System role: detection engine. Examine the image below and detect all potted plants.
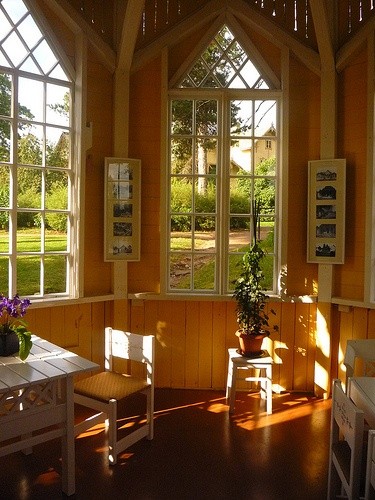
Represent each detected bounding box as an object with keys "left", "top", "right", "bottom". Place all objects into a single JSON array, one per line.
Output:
[{"left": 229, "top": 242, "right": 279, "bottom": 359}]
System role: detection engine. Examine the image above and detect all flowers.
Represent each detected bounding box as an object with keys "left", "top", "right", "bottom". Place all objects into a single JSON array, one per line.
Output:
[{"left": 0, "top": 292, "right": 33, "bottom": 361}]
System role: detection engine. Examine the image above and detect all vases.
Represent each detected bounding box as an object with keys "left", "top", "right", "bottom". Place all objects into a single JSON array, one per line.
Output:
[{"left": 0, "top": 332, "right": 19, "bottom": 357}]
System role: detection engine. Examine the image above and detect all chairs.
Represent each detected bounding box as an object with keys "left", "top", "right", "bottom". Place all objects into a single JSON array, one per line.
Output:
[
  {"left": 327, "top": 378, "right": 375, "bottom": 500},
  {"left": 344, "top": 339, "right": 375, "bottom": 441},
  {"left": 364, "top": 429, "right": 375, "bottom": 500},
  {"left": 73, "top": 327, "right": 155, "bottom": 466}
]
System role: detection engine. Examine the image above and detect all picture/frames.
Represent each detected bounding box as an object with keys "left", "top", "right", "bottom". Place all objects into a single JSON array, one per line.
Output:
[
  {"left": 104, "top": 156, "right": 141, "bottom": 262},
  {"left": 306, "top": 159, "right": 345, "bottom": 264}
]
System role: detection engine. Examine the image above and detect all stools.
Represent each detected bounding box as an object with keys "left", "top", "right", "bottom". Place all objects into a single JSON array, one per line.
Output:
[{"left": 226, "top": 348, "right": 273, "bottom": 416}]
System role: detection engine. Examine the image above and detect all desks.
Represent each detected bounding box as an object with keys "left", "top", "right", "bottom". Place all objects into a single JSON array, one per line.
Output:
[
  {"left": 0, "top": 334, "right": 100, "bottom": 496},
  {"left": 341, "top": 376, "right": 375, "bottom": 496}
]
